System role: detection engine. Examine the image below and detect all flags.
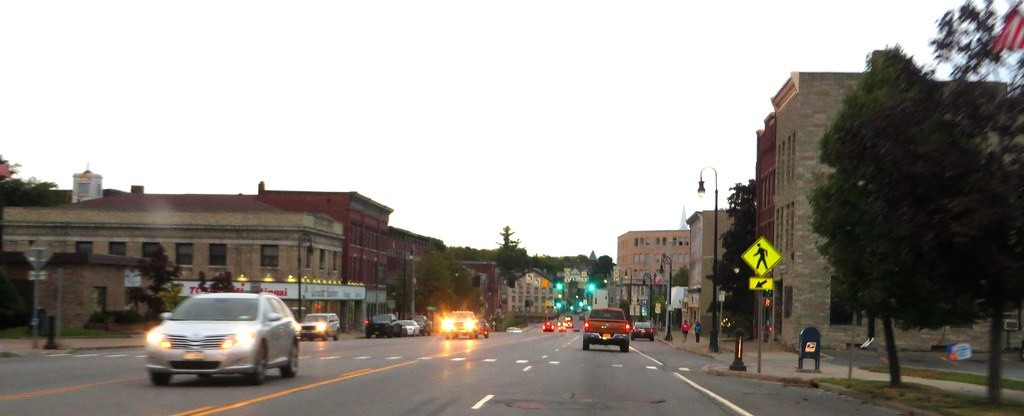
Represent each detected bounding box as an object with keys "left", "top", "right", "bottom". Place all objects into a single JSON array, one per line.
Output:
[{"left": 987, "top": 10, "right": 1024, "bottom": 53}]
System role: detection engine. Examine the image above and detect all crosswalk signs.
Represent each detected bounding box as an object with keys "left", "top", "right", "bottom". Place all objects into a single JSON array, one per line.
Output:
[{"left": 742, "top": 235, "right": 782, "bottom": 278}]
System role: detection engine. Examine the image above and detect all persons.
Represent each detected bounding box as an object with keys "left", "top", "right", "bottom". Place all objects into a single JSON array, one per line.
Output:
[
  {"left": 694, "top": 321, "right": 702, "bottom": 343},
  {"left": 680, "top": 320, "right": 690, "bottom": 344}
]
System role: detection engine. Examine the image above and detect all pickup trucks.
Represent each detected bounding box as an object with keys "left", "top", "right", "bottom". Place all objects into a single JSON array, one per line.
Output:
[{"left": 581, "top": 307, "right": 630, "bottom": 352}]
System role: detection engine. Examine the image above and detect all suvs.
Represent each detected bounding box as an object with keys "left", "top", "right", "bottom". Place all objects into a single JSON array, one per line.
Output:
[{"left": 299, "top": 312, "right": 340, "bottom": 341}]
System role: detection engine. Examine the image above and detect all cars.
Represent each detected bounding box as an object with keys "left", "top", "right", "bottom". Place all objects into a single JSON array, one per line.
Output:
[
  {"left": 142, "top": 289, "right": 300, "bottom": 384},
  {"left": 443, "top": 311, "right": 477, "bottom": 338},
  {"left": 505, "top": 305, "right": 593, "bottom": 334},
  {"left": 631, "top": 321, "right": 653, "bottom": 339},
  {"left": 397, "top": 318, "right": 420, "bottom": 336},
  {"left": 474, "top": 319, "right": 489, "bottom": 337},
  {"left": 411, "top": 316, "right": 432, "bottom": 336},
  {"left": 364, "top": 312, "right": 402, "bottom": 338}
]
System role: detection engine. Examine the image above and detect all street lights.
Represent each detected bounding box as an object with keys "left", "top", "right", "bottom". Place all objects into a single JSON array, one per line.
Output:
[
  {"left": 293, "top": 232, "right": 314, "bottom": 323},
  {"left": 641, "top": 272, "right": 651, "bottom": 321},
  {"left": 698, "top": 166, "right": 720, "bottom": 354},
  {"left": 623, "top": 266, "right": 632, "bottom": 307},
  {"left": 658, "top": 256, "right": 673, "bottom": 341}
]
